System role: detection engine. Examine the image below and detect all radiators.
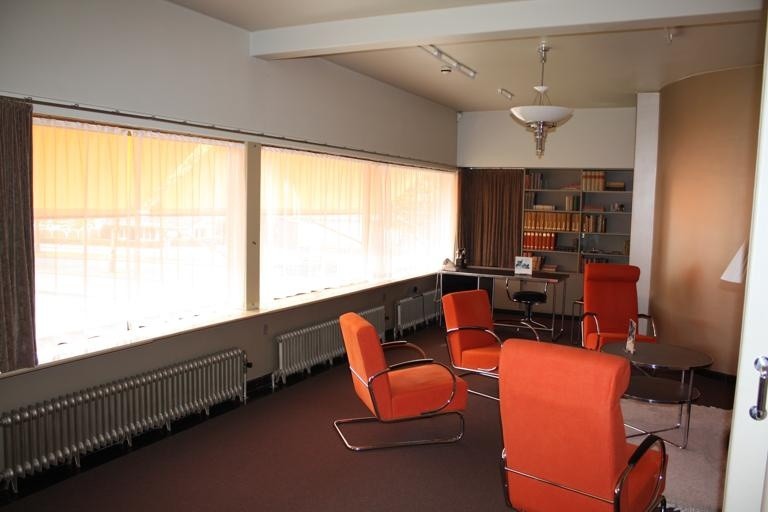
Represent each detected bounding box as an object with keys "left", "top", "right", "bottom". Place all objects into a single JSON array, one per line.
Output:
[
  {"left": 1, "top": 347, "right": 252, "bottom": 493},
  {"left": 271, "top": 306, "right": 385, "bottom": 390},
  {"left": 394, "top": 289, "right": 440, "bottom": 339}
]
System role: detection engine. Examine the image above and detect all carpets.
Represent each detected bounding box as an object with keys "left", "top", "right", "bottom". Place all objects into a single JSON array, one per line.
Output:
[{"left": 620, "top": 398, "right": 733, "bottom": 512}]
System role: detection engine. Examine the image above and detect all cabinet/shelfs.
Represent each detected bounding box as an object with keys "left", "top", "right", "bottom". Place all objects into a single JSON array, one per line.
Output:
[{"left": 492, "top": 167, "right": 634, "bottom": 317}]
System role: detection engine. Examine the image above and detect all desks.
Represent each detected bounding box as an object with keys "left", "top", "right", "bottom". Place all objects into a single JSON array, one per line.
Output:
[
  {"left": 600, "top": 342, "right": 714, "bottom": 449},
  {"left": 440, "top": 266, "right": 569, "bottom": 341}
]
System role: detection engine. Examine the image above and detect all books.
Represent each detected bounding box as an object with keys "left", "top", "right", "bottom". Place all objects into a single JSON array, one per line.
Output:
[{"left": 522, "top": 167, "right": 630, "bottom": 275}]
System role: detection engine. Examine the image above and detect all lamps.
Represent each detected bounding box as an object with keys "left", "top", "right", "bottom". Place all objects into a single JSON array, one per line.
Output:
[{"left": 510, "top": 42, "right": 573, "bottom": 158}]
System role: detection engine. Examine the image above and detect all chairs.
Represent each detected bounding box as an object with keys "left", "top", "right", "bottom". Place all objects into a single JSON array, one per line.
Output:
[
  {"left": 499, "top": 338, "right": 669, "bottom": 511},
  {"left": 570, "top": 295, "right": 584, "bottom": 346},
  {"left": 333, "top": 312, "right": 467, "bottom": 452},
  {"left": 581, "top": 263, "right": 657, "bottom": 352},
  {"left": 442, "top": 290, "right": 502, "bottom": 401},
  {"left": 506, "top": 278, "right": 548, "bottom": 341}
]
